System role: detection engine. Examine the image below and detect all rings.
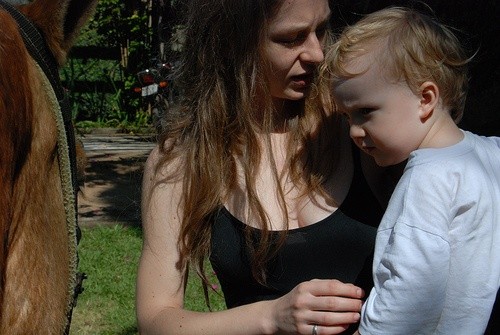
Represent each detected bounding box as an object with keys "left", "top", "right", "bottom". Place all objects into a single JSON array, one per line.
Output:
[{"left": 313, "top": 324, "right": 317, "bottom": 335}]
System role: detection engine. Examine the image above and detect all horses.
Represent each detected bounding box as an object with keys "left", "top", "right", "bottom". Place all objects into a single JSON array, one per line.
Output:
[{"left": 0, "top": 0, "right": 94, "bottom": 334}]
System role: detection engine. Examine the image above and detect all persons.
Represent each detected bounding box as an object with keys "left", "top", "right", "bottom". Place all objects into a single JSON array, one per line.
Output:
[
  {"left": 331, "top": 7, "right": 499, "bottom": 335},
  {"left": 134, "top": 0, "right": 398, "bottom": 335}
]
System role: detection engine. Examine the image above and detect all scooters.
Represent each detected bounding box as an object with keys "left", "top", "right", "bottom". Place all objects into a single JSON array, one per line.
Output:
[{"left": 134, "top": 57, "right": 170, "bottom": 147}]
